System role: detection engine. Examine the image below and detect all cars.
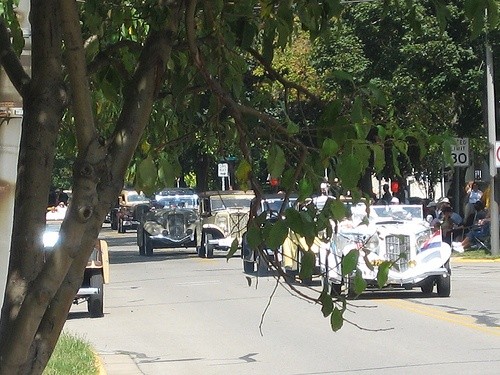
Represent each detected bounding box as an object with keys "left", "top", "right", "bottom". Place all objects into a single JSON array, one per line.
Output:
[{"left": 316, "top": 203, "right": 452, "bottom": 299}]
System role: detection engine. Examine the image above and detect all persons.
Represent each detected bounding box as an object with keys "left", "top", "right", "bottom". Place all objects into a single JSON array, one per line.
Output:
[
  {"left": 467, "top": 182, "right": 483, "bottom": 205},
  {"left": 48, "top": 188, "right": 57, "bottom": 207},
  {"left": 425, "top": 200, "right": 443, "bottom": 226},
  {"left": 59, "top": 188, "right": 68, "bottom": 206},
  {"left": 437, "top": 196, "right": 454, "bottom": 210},
  {"left": 452, "top": 201, "right": 490, "bottom": 253},
  {"left": 382, "top": 183, "right": 392, "bottom": 205},
  {"left": 442, "top": 206, "right": 464, "bottom": 244},
  {"left": 396, "top": 184, "right": 405, "bottom": 204}
]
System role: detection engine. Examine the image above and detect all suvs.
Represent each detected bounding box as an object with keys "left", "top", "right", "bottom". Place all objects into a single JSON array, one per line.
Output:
[
  {"left": 131, "top": 188, "right": 207, "bottom": 258},
  {"left": 194, "top": 191, "right": 259, "bottom": 260},
  {"left": 40, "top": 201, "right": 111, "bottom": 318},
  {"left": 101, "top": 190, "right": 150, "bottom": 234},
  {"left": 243, "top": 197, "right": 374, "bottom": 283}
]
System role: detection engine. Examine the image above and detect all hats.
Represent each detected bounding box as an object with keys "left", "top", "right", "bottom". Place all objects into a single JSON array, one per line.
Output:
[
  {"left": 441, "top": 198, "right": 450, "bottom": 203},
  {"left": 391, "top": 197, "right": 399, "bottom": 203},
  {"left": 426, "top": 202, "right": 436, "bottom": 207}
]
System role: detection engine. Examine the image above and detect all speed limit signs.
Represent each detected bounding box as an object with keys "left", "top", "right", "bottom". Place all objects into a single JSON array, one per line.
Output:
[{"left": 447, "top": 138, "right": 470, "bottom": 168}]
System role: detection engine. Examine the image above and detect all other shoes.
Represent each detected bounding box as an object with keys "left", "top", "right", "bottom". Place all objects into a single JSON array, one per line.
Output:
[{"left": 452, "top": 242, "right": 464, "bottom": 253}]
